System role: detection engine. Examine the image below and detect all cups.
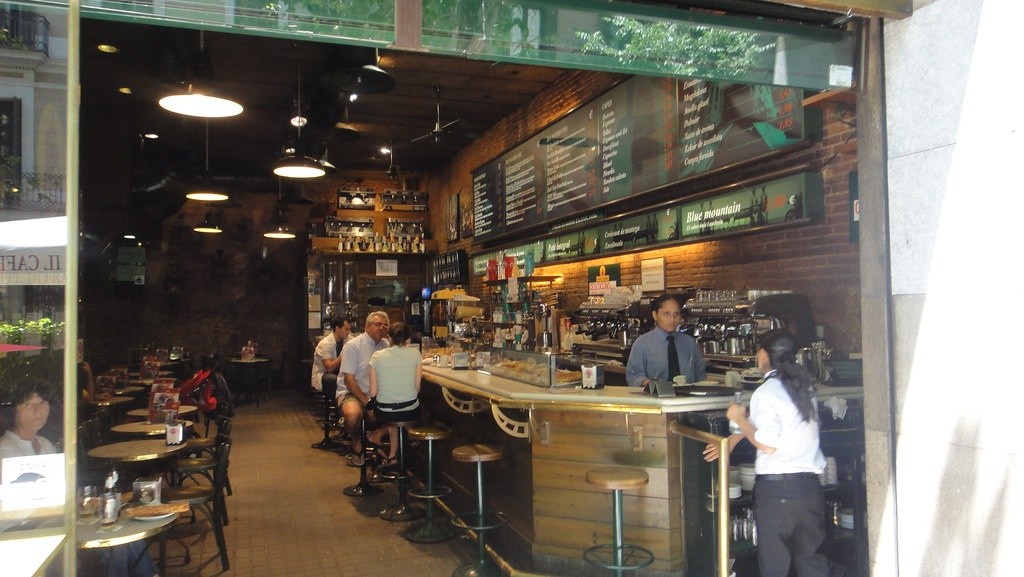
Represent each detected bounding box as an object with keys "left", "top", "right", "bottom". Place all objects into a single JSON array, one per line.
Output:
[{"left": 77, "top": 485, "right": 104, "bottom": 523}]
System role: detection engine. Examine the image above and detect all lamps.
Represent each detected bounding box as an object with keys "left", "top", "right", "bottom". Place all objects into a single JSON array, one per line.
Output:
[{"left": 94, "top": 20, "right": 395, "bottom": 240}]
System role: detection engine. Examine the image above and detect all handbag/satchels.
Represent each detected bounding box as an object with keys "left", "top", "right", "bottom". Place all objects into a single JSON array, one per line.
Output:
[{"left": 365, "top": 395, "right": 417, "bottom": 411}]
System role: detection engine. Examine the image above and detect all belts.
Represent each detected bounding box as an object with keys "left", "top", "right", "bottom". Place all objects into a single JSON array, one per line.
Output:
[{"left": 756, "top": 472, "right": 819, "bottom": 481}]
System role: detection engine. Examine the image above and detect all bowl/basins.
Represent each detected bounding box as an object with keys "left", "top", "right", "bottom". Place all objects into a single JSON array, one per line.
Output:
[{"left": 728, "top": 462, "right": 756, "bottom": 500}]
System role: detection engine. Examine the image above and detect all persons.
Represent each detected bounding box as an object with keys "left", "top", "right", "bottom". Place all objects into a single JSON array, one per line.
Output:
[
  {"left": 0, "top": 381, "right": 161, "bottom": 577},
  {"left": 369, "top": 321, "right": 423, "bottom": 472},
  {"left": 626, "top": 293, "right": 708, "bottom": 386},
  {"left": 189, "top": 352, "right": 234, "bottom": 420},
  {"left": 162, "top": 352, "right": 193, "bottom": 382},
  {"left": 24, "top": 341, "right": 111, "bottom": 422},
  {"left": 311, "top": 314, "right": 354, "bottom": 393},
  {"left": 335, "top": 309, "right": 391, "bottom": 466},
  {"left": 701, "top": 328, "right": 836, "bottom": 577}
]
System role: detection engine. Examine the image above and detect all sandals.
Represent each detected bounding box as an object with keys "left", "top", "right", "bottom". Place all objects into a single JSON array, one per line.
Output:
[{"left": 377, "top": 456, "right": 400, "bottom": 471}]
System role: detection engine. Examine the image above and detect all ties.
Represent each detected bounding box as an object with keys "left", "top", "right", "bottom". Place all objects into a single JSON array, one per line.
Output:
[{"left": 666, "top": 335, "right": 680, "bottom": 381}]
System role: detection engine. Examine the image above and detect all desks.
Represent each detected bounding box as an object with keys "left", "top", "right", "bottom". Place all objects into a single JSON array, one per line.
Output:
[
  {"left": 229, "top": 357, "right": 270, "bottom": 407},
  {"left": 75, "top": 367, "right": 203, "bottom": 577}
]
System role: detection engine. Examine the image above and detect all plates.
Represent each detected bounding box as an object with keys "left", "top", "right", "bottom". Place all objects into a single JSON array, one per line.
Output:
[
  {"left": 838, "top": 508, "right": 868, "bottom": 531},
  {"left": 133, "top": 511, "right": 174, "bottom": 521}
]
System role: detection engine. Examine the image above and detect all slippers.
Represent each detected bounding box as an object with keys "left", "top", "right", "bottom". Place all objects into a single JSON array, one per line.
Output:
[
  {"left": 351, "top": 448, "right": 366, "bottom": 466},
  {"left": 364, "top": 435, "right": 389, "bottom": 460}
]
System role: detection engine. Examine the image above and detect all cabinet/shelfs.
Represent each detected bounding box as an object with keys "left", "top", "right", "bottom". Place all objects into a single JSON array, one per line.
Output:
[
  {"left": 481, "top": 274, "right": 560, "bottom": 348},
  {"left": 329, "top": 186, "right": 427, "bottom": 239}
]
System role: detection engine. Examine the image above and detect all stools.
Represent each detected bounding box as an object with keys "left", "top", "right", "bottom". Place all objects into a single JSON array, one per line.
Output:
[
  {"left": 312, "top": 387, "right": 513, "bottom": 577},
  {"left": 583, "top": 466, "right": 655, "bottom": 577}
]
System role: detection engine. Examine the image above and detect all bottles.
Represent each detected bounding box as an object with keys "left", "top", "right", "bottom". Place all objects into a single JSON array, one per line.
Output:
[
  {"left": 728, "top": 514, "right": 757, "bottom": 547},
  {"left": 337, "top": 233, "right": 426, "bottom": 254},
  {"left": 729, "top": 392, "right": 746, "bottom": 434},
  {"left": 490, "top": 282, "right": 556, "bottom": 355},
  {"left": 100, "top": 470, "right": 123, "bottom": 530}
]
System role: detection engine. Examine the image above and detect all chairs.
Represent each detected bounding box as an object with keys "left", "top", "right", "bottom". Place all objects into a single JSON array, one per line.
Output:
[{"left": 158, "top": 389, "right": 232, "bottom": 572}]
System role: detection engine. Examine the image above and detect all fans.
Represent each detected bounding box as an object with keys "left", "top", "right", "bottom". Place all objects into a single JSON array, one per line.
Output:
[{"left": 410, "top": 84, "right": 465, "bottom": 145}]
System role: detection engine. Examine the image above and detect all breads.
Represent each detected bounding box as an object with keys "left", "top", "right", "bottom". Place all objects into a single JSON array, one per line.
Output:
[{"left": 493, "top": 359, "right": 583, "bottom": 382}]
